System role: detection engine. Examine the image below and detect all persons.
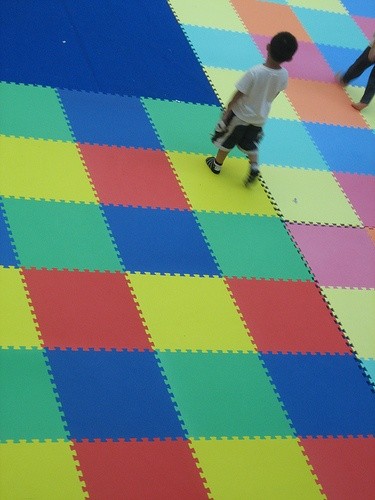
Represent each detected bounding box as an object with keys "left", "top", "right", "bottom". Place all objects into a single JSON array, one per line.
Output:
[
  {"left": 335, "top": 29, "right": 374, "bottom": 111},
  {"left": 206, "top": 31, "right": 299, "bottom": 183}
]
